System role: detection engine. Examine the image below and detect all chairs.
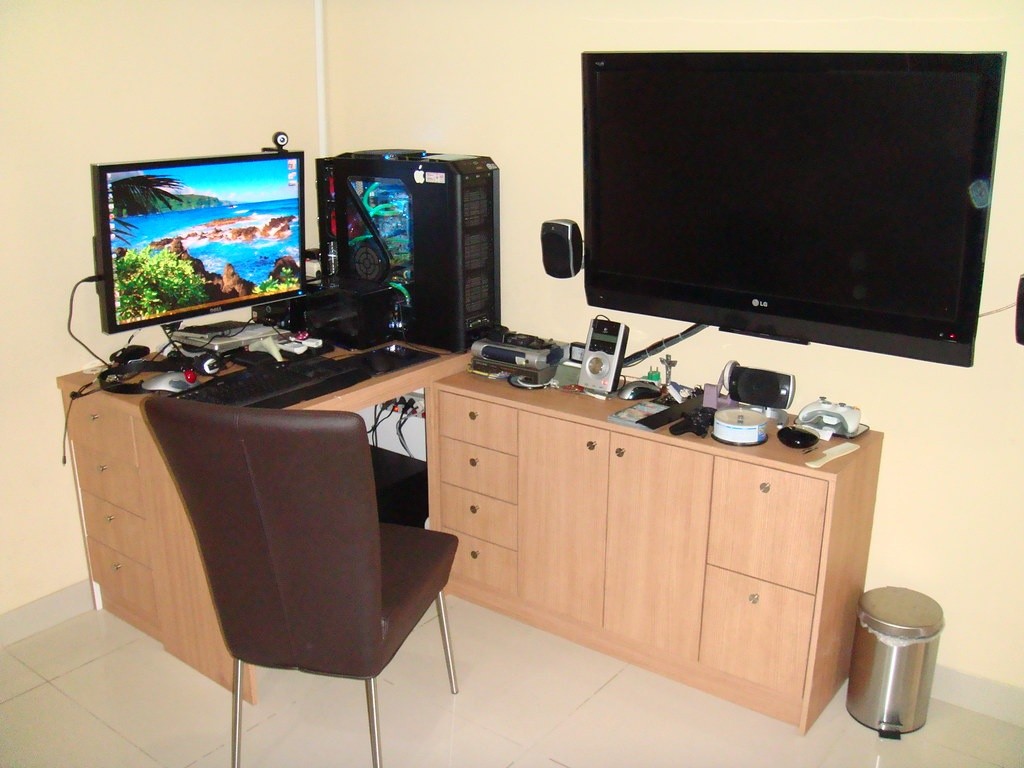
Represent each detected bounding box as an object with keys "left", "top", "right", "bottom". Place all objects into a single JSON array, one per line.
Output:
[{"left": 141, "top": 396, "right": 459, "bottom": 768}]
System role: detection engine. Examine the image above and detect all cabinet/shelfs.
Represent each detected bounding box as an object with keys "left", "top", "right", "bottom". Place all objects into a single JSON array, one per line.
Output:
[{"left": 56, "top": 336, "right": 884, "bottom": 738}]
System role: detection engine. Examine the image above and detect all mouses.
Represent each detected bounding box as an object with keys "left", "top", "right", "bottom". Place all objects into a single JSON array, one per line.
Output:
[
  {"left": 365, "top": 351, "right": 394, "bottom": 372},
  {"left": 618, "top": 380, "right": 662, "bottom": 401},
  {"left": 140, "top": 371, "right": 200, "bottom": 395}
]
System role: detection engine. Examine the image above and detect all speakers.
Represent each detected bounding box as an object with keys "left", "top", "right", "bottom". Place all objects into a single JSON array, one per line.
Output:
[
  {"left": 729, "top": 367, "right": 795, "bottom": 429},
  {"left": 541, "top": 219, "right": 583, "bottom": 279}
]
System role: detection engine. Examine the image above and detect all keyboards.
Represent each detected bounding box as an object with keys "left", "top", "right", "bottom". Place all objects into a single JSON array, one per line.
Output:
[{"left": 176, "top": 356, "right": 348, "bottom": 406}]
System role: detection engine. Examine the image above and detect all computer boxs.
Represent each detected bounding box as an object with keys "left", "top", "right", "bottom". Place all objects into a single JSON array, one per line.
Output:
[{"left": 316, "top": 149, "right": 500, "bottom": 354}]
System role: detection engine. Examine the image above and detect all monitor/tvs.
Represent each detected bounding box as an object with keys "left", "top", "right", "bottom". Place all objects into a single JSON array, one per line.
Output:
[
  {"left": 581, "top": 51, "right": 1006, "bottom": 367},
  {"left": 90, "top": 152, "right": 306, "bottom": 358}
]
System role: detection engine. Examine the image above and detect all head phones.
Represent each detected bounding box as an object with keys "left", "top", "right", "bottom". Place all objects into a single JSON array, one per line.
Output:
[{"left": 191, "top": 353, "right": 235, "bottom": 376}]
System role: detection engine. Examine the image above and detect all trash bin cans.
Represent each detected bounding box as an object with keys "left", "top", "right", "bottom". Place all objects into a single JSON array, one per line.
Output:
[{"left": 844, "top": 585, "right": 945, "bottom": 739}]
